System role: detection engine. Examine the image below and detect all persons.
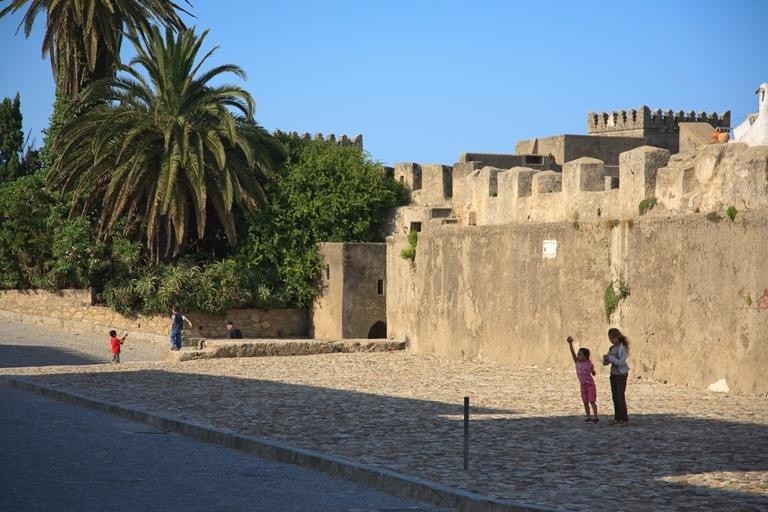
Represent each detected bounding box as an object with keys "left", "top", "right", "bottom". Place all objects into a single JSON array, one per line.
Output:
[
  {"left": 110, "top": 330, "right": 128, "bottom": 363},
  {"left": 224, "top": 321, "right": 243, "bottom": 338},
  {"left": 567, "top": 336, "right": 599, "bottom": 422},
  {"left": 169, "top": 305, "right": 193, "bottom": 351},
  {"left": 602, "top": 328, "right": 629, "bottom": 427}
]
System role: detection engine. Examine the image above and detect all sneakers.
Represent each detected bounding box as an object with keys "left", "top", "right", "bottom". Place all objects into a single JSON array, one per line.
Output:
[{"left": 585, "top": 417, "right": 628, "bottom": 428}]
